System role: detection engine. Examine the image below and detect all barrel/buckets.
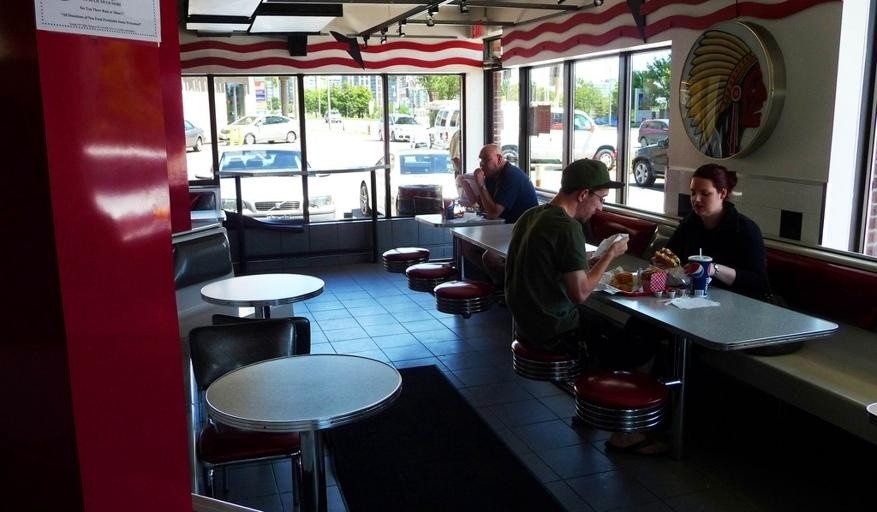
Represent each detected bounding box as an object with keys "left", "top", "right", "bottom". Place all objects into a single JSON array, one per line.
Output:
[{"left": 397, "top": 184, "right": 443, "bottom": 217}]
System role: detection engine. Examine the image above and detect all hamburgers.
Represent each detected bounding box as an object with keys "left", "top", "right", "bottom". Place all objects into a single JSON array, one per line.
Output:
[
  {"left": 652, "top": 248, "right": 681, "bottom": 268},
  {"left": 608, "top": 272, "right": 633, "bottom": 292}
]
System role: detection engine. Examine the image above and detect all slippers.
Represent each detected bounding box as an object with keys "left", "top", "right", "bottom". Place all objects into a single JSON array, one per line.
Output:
[{"left": 605, "top": 436, "right": 657, "bottom": 455}]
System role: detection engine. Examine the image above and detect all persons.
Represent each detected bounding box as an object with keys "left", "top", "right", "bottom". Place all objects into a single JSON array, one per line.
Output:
[
  {"left": 505, "top": 157, "right": 655, "bottom": 452},
  {"left": 456, "top": 144, "right": 538, "bottom": 224},
  {"left": 686, "top": 31, "right": 767, "bottom": 158},
  {"left": 624, "top": 163, "right": 769, "bottom": 371}
]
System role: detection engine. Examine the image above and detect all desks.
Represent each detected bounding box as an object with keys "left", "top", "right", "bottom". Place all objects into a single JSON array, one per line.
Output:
[
  {"left": 199, "top": 272, "right": 324, "bottom": 319},
  {"left": 205, "top": 353, "right": 403, "bottom": 512}
]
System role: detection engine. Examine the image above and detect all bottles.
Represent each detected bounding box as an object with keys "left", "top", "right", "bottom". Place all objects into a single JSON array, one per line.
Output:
[{"left": 535, "top": 165, "right": 541, "bottom": 187}]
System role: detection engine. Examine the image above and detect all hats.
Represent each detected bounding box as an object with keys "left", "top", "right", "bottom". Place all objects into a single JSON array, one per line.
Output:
[{"left": 561, "top": 159, "right": 625, "bottom": 188}]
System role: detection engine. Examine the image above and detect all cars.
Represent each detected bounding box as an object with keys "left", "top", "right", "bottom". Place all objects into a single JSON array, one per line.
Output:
[
  {"left": 220, "top": 113, "right": 299, "bottom": 145},
  {"left": 427, "top": 98, "right": 669, "bottom": 188},
  {"left": 193, "top": 146, "right": 337, "bottom": 223},
  {"left": 359, "top": 150, "right": 459, "bottom": 219},
  {"left": 324, "top": 109, "right": 342, "bottom": 122},
  {"left": 377, "top": 114, "right": 423, "bottom": 142},
  {"left": 184, "top": 120, "right": 206, "bottom": 154}
]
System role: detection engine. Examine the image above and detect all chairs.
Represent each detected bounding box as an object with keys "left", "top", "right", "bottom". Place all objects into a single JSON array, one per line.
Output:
[
  {"left": 394, "top": 183, "right": 455, "bottom": 257},
  {"left": 188, "top": 322, "right": 305, "bottom": 511},
  {"left": 170, "top": 221, "right": 294, "bottom": 349},
  {"left": 209, "top": 313, "right": 310, "bottom": 355},
  {"left": 188, "top": 179, "right": 222, "bottom": 229}
]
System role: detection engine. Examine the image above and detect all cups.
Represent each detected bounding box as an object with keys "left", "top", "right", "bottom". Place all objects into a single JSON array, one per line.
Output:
[
  {"left": 654, "top": 288, "right": 686, "bottom": 299},
  {"left": 688, "top": 255, "right": 713, "bottom": 296},
  {"left": 443, "top": 199, "right": 454, "bottom": 220}
]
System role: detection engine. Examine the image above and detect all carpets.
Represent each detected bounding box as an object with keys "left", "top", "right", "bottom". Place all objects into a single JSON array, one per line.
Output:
[{"left": 324, "top": 364, "right": 566, "bottom": 512}]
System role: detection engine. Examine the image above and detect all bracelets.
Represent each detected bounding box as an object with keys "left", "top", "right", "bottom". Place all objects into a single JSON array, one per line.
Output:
[
  {"left": 714, "top": 263, "right": 719, "bottom": 278},
  {"left": 479, "top": 184, "right": 487, "bottom": 192}
]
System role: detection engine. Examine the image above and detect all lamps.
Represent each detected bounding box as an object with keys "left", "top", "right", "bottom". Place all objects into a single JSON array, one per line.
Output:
[
  {"left": 356, "top": 1, "right": 471, "bottom": 48},
  {"left": 246, "top": 1, "right": 343, "bottom": 34}
]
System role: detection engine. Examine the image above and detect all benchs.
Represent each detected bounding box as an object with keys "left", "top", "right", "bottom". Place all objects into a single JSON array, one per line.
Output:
[{"left": 544, "top": 195, "right": 877, "bottom": 447}]
